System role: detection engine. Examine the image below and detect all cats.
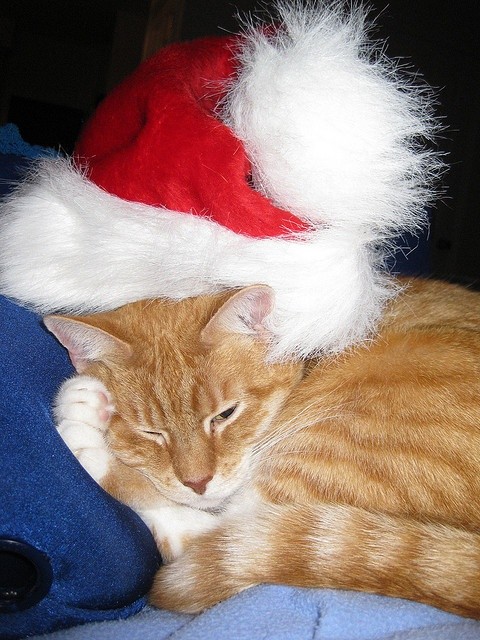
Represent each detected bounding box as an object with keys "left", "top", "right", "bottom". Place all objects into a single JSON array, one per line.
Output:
[{"left": 41, "top": 275, "right": 479, "bottom": 627}]
